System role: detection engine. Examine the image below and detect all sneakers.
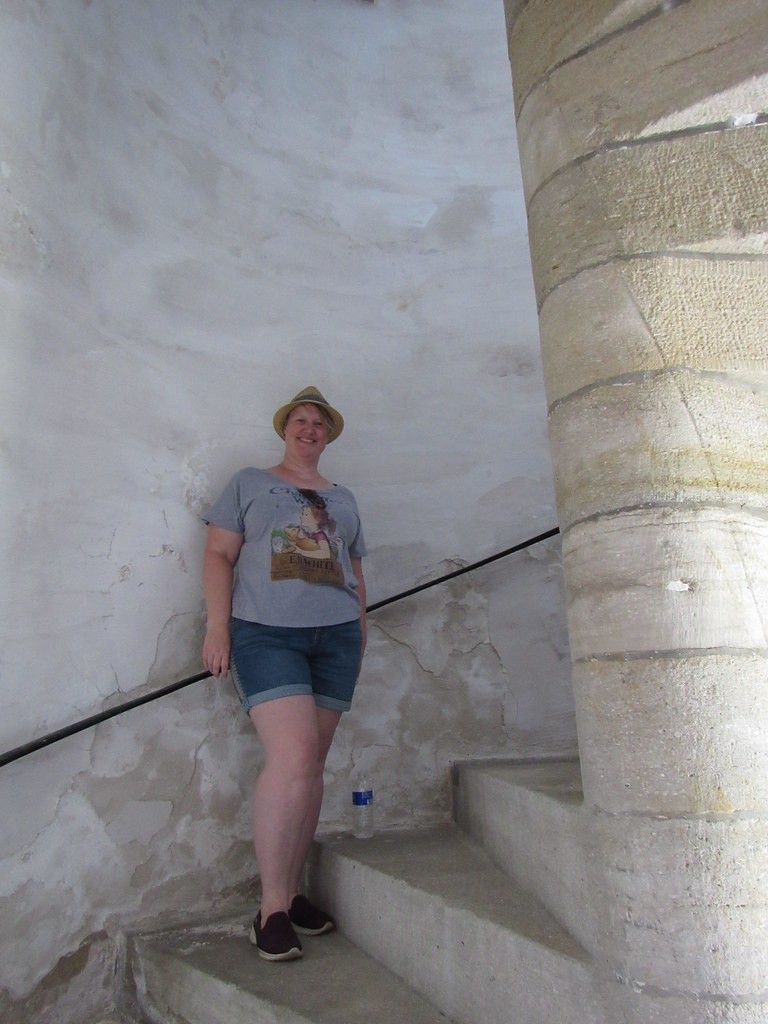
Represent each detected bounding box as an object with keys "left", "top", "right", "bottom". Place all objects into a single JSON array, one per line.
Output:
[
  {"left": 249, "top": 909, "right": 303, "bottom": 960},
  {"left": 288, "top": 893, "right": 334, "bottom": 935}
]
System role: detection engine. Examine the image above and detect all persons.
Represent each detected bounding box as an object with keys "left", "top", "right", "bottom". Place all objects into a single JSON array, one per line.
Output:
[{"left": 201, "top": 387, "right": 367, "bottom": 960}]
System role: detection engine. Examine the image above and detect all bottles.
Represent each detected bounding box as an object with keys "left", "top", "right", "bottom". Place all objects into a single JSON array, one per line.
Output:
[{"left": 351, "top": 770, "right": 375, "bottom": 839}]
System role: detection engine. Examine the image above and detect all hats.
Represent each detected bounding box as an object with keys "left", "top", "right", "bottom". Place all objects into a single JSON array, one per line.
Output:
[{"left": 273, "top": 386, "right": 344, "bottom": 444}]
